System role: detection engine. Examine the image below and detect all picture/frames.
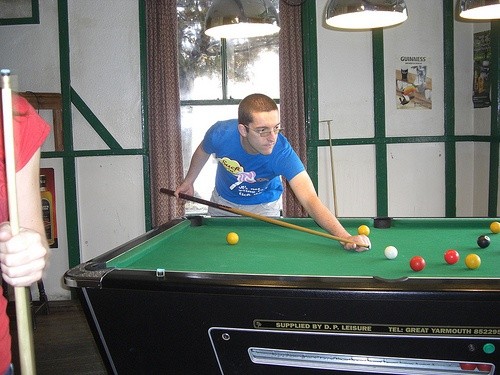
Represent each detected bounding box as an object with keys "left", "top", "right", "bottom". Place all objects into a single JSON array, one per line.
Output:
[{"left": 0, "top": 0, "right": 40, "bottom": 26}]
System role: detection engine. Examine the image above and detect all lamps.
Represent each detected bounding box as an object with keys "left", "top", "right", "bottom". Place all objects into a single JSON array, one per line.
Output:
[
  {"left": 454, "top": 0, "right": 500, "bottom": 25},
  {"left": 203, "top": 0, "right": 280, "bottom": 39},
  {"left": 321, "top": 0, "right": 409, "bottom": 32}
]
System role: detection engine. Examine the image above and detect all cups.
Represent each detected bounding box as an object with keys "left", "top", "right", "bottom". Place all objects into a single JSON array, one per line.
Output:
[
  {"left": 425, "top": 90, "right": 431, "bottom": 99},
  {"left": 407, "top": 71, "right": 417, "bottom": 83},
  {"left": 401, "top": 68, "right": 408, "bottom": 79},
  {"left": 414, "top": 81, "right": 423, "bottom": 92}
]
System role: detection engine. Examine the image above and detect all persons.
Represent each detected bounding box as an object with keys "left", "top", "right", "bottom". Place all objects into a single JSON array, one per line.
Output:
[
  {"left": 175, "top": 93, "right": 371, "bottom": 252},
  {"left": 0, "top": 86, "right": 51, "bottom": 375}
]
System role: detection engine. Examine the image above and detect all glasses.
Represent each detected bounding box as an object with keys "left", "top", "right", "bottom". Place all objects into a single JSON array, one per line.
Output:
[{"left": 244, "top": 125, "right": 284, "bottom": 138}]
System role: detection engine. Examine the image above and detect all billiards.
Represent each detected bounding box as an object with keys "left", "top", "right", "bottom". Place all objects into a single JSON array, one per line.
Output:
[
  {"left": 477, "top": 234, "right": 490, "bottom": 249},
  {"left": 464, "top": 253, "right": 481, "bottom": 269},
  {"left": 383, "top": 245, "right": 398, "bottom": 259},
  {"left": 409, "top": 255, "right": 425, "bottom": 271},
  {"left": 226, "top": 231, "right": 240, "bottom": 246},
  {"left": 357, "top": 224, "right": 370, "bottom": 236},
  {"left": 489, "top": 221, "right": 500, "bottom": 234},
  {"left": 444, "top": 249, "right": 459, "bottom": 264}
]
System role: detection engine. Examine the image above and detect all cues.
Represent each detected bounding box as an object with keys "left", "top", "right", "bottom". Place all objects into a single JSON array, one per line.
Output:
[
  {"left": 158, "top": 188, "right": 372, "bottom": 250},
  {"left": 0, "top": 68, "right": 36, "bottom": 375}
]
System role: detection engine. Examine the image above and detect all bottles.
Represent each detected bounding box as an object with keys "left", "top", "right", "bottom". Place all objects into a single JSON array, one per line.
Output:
[
  {"left": 39, "top": 175, "right": 54, "bottom": 246},
  {"left": 417, "top": 65, "right": 425, "bottom": 85},
  {"left": 478, "top": 61, "right": 490, "bottom": 94}
]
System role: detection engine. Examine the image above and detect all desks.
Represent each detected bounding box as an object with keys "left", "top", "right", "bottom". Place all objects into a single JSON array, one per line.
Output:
[{"left": 63, "top": 214, "right": 500, "bottom": 375}]
